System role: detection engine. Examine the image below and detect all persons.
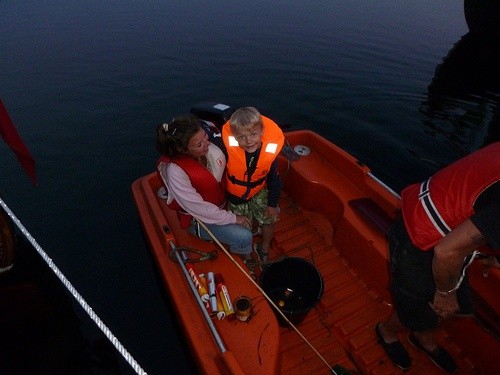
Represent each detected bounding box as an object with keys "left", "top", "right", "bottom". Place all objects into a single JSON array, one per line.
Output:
[
  {"left": 156, "top": 116, "right": 257, "bottom": 268},
  {"left": 374, "top": 139, "right": 500, "bottom": 372},
  {"left": 214, "top": 105, "right": 286, "bottom": 271}
]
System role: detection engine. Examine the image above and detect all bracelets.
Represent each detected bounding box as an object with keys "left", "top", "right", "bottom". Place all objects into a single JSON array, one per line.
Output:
[{"left": 436, "top": 288, "right": 456, "bottom": 296}]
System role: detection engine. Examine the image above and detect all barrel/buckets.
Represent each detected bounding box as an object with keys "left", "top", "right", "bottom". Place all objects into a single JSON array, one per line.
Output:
[{"left": 259, "top": 256, "right": 324, "bottom": 328}]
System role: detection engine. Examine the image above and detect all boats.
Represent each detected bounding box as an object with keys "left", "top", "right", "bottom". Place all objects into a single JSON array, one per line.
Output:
[{"left": 130, "top": 129, "right": 500, "bottom": 375}]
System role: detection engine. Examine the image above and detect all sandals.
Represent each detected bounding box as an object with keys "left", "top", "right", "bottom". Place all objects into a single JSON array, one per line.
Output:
[{"left": 253, "top": 242, "right": 269, "bottom": 272}]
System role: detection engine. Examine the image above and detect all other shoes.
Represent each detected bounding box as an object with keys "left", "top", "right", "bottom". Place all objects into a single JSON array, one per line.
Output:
[
  {"left": 407, "top": 331, "right": 458, "bottom": 373},
  {"left": 376, "top": 321, "right": 413, "bottom": 370},
  {"left": 242, "top": 258, "right": 257, "bottom": 280}
]
